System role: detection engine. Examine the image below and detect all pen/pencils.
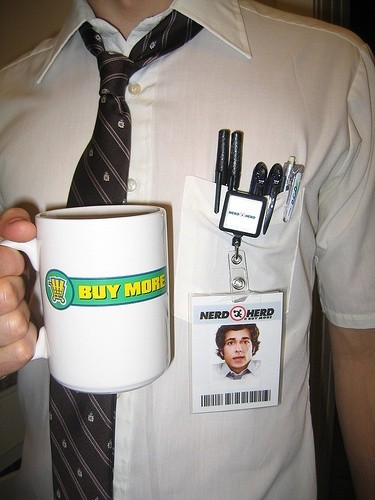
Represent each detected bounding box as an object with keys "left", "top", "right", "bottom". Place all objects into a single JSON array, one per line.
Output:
[
  {"left": 214, "top": 129, "right": 243, "bottom": 214},
  {"left": 262, "top": 163, "right": 283, "bottom": 236},
  {"left": 281, "top": 155, "right": 305, "bottom": 222},
  {"left": 249, "top": 162, "right": 267, "bottom": 196}
]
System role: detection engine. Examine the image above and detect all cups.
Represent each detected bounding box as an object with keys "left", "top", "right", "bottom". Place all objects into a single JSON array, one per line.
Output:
[{"left": 0, "top": 206, "right": 170, "bottom": 395}]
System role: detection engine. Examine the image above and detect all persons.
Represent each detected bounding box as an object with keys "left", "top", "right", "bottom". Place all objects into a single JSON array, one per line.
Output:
[
  {"left": 206, "top": 323, "right": 274, "bottom": 390},
  {"left": 0, "top": 0, "right": 374, "bottom": 499}
]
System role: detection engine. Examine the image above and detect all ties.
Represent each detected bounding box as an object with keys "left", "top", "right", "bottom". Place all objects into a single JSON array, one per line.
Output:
[
  {"left": 228, "top": 369, "right": 251, "bottom": 380},
  {"left": 49, "top": 9, "right": 204, "bottom": 500}
]
